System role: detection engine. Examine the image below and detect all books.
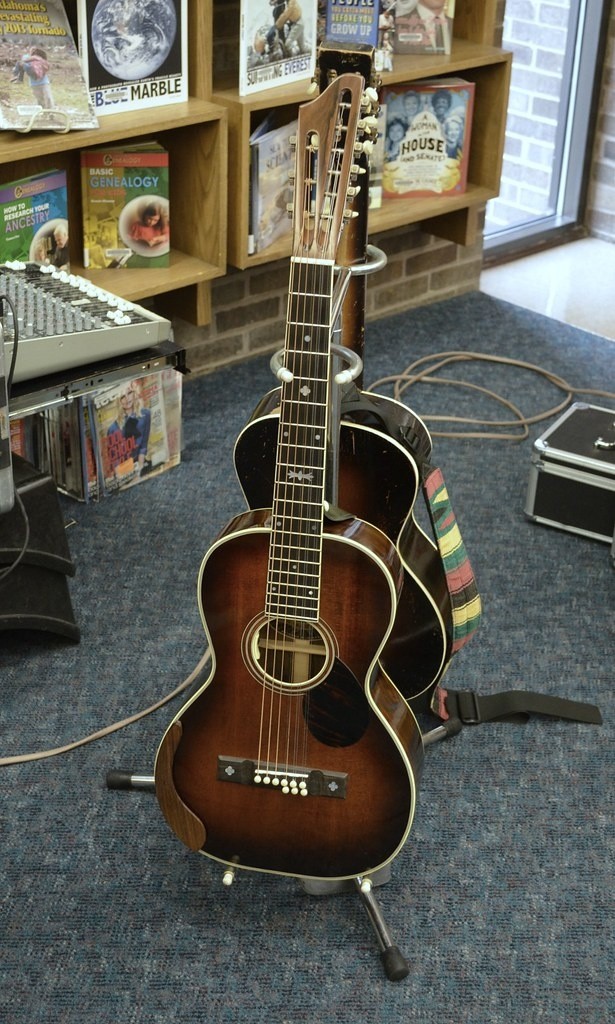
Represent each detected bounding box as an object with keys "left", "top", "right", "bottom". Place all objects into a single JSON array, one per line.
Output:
[
  {"left": 77, "top": 0, "right": 188, "bottom": 116},
  {"left": 238, "top": 0, "right": 318, "bottom": 252},
  {"left": 32, "top": 368, "right": 181, "bottom": 498},
  {"left": 380, "top": 0, "right": 476, "bottom": 202},
  {"left": 0, "top": 141, "right": 170, "bottom": 272},
  {"left": 0, "top": 0, "right": 100, "bottom": 132}
]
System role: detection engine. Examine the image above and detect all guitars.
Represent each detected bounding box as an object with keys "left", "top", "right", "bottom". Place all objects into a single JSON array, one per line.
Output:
[
  {"left": 229, "top": 35, "right": 454, "bottom": 700},
  {"left": 150, "top": 68, "right": 428, "bottom": 883}
]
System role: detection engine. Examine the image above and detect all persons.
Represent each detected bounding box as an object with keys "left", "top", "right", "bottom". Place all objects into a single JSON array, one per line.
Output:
[
  {"left": 256, "top": 0, "right": 465, "bottom": 226},
  {"left": 130, "top": 202, "right": 165, "bottom": 251},
  {"left": 9, "top": 44, "right": 56, "bottom": 122},
  {"left": 37, "top": 226, "right": 69, "bottom": 271},
  {"left": 106, "top": 380, "right": 151, "bottom": 488}
]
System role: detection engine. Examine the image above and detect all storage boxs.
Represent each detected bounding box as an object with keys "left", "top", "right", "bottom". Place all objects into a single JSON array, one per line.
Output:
[{"left": 524, "top": 401, "right": 615, "bottom": 543}]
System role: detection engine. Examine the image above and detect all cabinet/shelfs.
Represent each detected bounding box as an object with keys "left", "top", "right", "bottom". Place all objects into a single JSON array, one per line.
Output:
[{"left": 1, "top": 0, "right": 513, "bottom": 383}]
